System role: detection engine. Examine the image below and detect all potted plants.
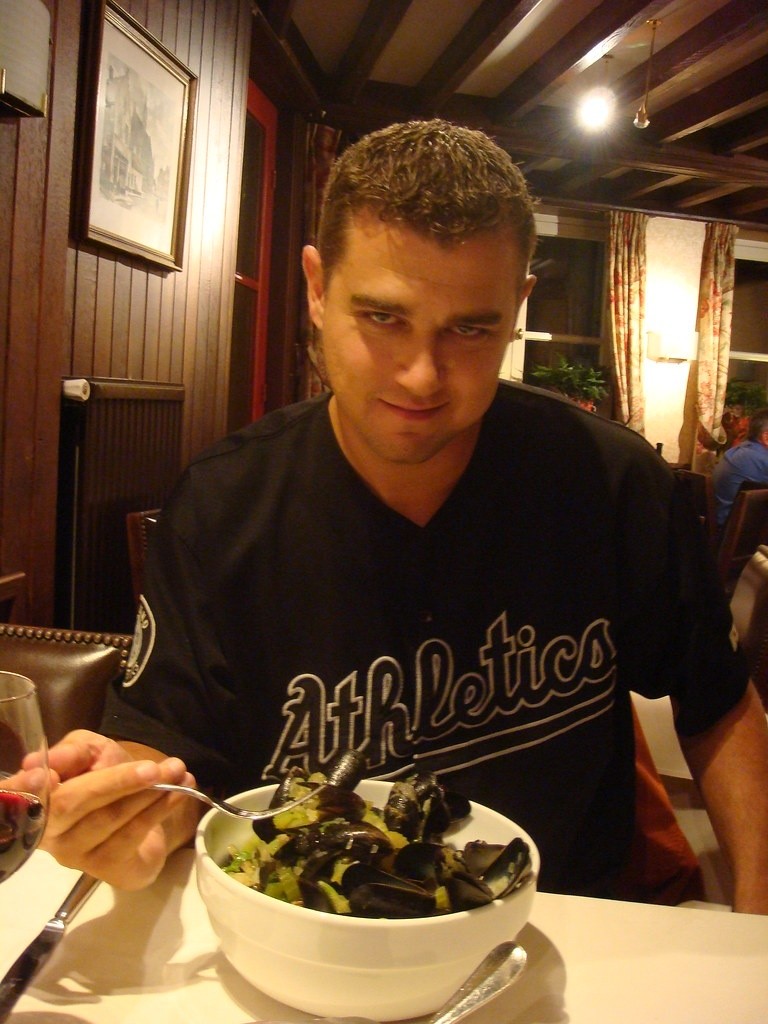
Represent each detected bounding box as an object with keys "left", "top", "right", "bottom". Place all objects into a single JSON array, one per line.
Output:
[
  {"left": 722, "top": 372, "right": 768, "bottom": 451},
  {"left": 532, "top": 352, "right": 612, "bottom": 412}
]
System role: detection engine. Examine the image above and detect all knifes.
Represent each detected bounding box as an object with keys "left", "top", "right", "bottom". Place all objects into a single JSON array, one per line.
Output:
[{"left": 0, "top": 871, "right": 99, "bottom": 1022}]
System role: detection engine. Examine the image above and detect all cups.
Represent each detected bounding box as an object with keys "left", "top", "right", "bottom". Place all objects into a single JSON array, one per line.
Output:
[{"left": 0, "top": 670, "right": 49, "bottom": 889}]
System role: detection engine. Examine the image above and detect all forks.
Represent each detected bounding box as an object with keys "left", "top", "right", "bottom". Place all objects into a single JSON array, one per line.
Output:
[{"left": 138, "top": 783, "right": 327, "bottom": 822}]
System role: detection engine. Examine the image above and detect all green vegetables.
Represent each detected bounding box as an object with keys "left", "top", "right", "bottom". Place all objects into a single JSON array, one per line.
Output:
[{"left": 222, "top": 772, "right": 453, "bottom": 915}]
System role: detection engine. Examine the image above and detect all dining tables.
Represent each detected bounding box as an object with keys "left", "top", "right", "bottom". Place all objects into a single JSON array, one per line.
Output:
[
  {"left": 0, "top": 847, "right": 768, "bottom": 1024},
  {"left": 74, "top": 0, "right": 201, "bottom": 272}
]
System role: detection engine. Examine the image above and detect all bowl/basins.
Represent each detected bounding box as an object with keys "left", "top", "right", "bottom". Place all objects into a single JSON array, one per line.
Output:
[{"left": 195, "top": 780, "right": 541, "bottom": 1022}]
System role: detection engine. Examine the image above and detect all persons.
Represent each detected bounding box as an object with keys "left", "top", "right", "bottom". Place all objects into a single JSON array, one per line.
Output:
[
  {"left": 710, "top": 408, "right": 768, "bottom": 529},
  {"left": 0, "top": 123, "right": 768, "bottom": 918}
]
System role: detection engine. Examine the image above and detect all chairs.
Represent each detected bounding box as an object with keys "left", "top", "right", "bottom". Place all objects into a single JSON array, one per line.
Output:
[{"left": 674, "top": 468, "right": 768, "bottom": 603}]
